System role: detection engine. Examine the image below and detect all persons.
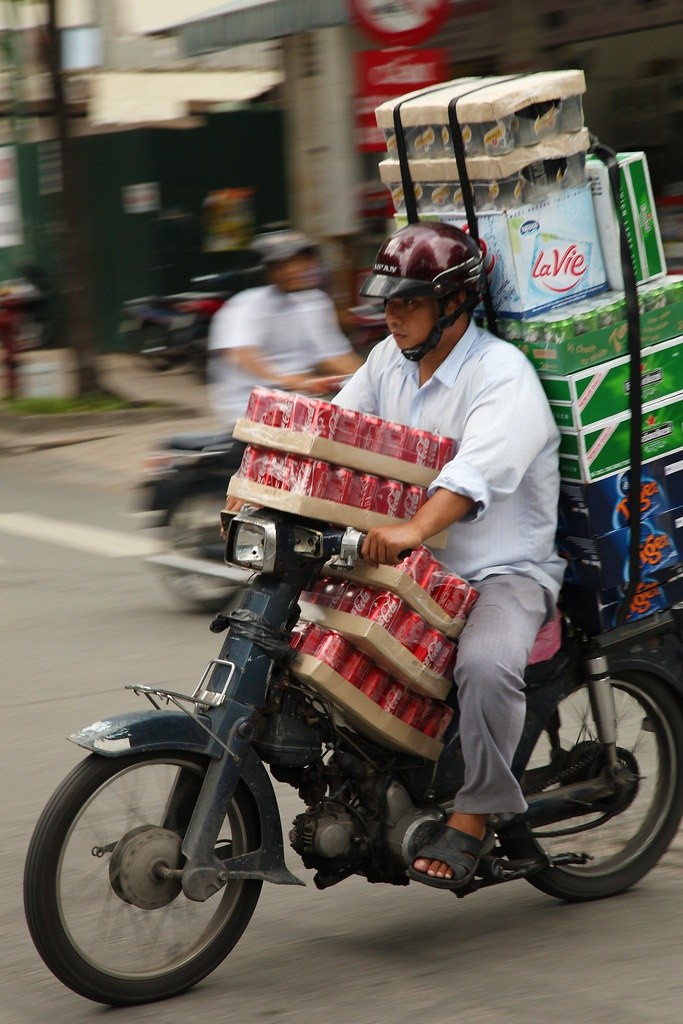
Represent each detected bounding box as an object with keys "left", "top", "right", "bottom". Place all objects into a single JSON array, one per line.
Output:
[
  {"left": 221, "top": 222, "right": 567, "bottom": 889},
  {"left": 0, "top": 265, "right": 98, "bottom": 393},
  {"left": 208, "top": 232, "right": 364, "bottom": 467}
]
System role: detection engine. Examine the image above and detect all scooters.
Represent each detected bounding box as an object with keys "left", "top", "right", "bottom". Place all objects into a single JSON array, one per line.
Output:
[{"left": 130, "top": 371, "right": 355, "bottom": 616}]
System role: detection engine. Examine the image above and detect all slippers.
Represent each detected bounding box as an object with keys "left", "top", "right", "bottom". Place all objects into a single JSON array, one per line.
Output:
[{"left": 407, "top": 818, "right": 496, "bottom": 889}]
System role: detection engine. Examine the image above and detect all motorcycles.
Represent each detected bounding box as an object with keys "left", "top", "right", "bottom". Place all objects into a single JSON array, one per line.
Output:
[{"left": 16, "top": 501, "right": 683, "bottom": 1004}]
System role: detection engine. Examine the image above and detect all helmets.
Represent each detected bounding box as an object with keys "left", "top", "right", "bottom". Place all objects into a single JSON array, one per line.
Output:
[{"left": 357, "top": 221, "right": 490, "bottom": 299}]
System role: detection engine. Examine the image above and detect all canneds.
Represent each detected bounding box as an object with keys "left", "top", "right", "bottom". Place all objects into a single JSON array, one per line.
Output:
[
  {"left": 476, "top": 274, "right": 683, "bottom": 343},
  {"left": 382, "top": 91, "right": 586, "bottom": 214},
  {"left": 287, "top": 541, "right": 480, "bottom": 743},
  {"left": 238, "top": 384, "right": 458, "bottom": 521}
]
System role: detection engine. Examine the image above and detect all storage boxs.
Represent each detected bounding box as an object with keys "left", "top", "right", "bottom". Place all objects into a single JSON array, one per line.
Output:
[
  {"left": 376, "top": 72, "right": 683, "bottom": 635},
  {"left": 227, "top": 419, "right": 466, "bottom": 760}
]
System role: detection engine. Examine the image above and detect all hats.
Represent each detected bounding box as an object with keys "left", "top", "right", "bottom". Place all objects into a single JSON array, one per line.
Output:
[{"left": 258, "top": 230, "right": 321, "bottom": 262}]
